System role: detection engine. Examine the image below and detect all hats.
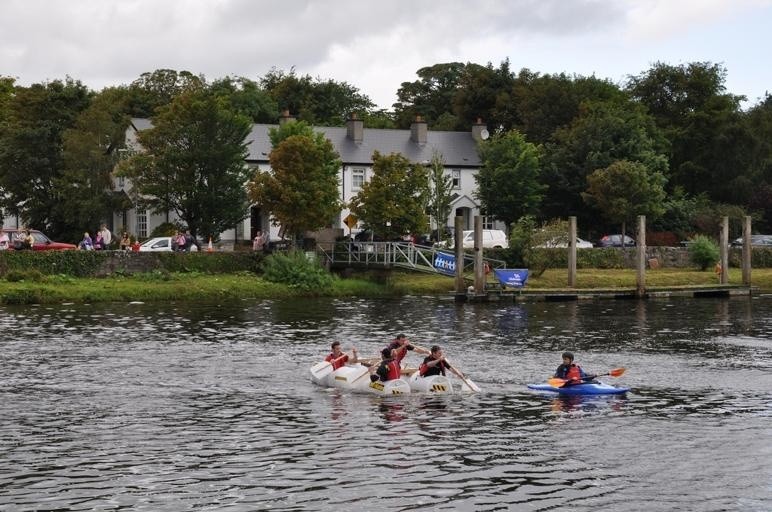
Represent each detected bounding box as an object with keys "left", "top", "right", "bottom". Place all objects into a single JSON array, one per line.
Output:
[{"left": 379, "top": 348, "right": 391, "bottom": 355}]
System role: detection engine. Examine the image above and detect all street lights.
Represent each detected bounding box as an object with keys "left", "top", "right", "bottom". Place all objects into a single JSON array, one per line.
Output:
[
  {"left": 116, "top": 146, "right": 138, "bottom": 237},
  {"left": 420, "top": 159, "right": 441, "bottom": 238}
]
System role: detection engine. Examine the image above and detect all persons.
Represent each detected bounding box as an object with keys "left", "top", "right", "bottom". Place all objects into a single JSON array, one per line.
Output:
[
  {"left": 419, "top": 346, "right": 466, "bottom": 380},
  {"left": 559, "top": 392, "right": 588, "bottom": 420},
  {"left": 368, "top": 347, "right": 401, "bottom": 383},
  {"left": 379, "top": 396, "right": 409, "bottom": 428},
  {"left": 261, "top": 227, "right": 271, "bottom": 252},
  {"left": 325, "top": 340, "right": 358, "bottom": 372},
  {"left": 13, "top": 227, "right": 26, "bottom": 251},
  {"left": 423, "top": 396, "right": 445, "bottom": 423},
  {"left": 78, "top": 223, "right": 196, "bottom": 253},
  {"left": 551, "top": 351, "right": 596, "bottom": 384},
  {"left": 387, "top": 334, "right": 432, "bottom": 372},
  {"left": 253, "top": 232, "right": 266, "bottom": 253},
  {"left": 329, "top": 385, "right": 352, "bottom": 419},
  {"left": 0, "top": 229, "right": 11, "bottom": 251},
  {"left": 24, "top": 230, "right": 35, "bottom": 251}
]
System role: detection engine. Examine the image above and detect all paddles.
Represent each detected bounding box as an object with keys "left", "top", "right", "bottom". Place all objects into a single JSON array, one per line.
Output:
[{"left": 547, "top": 368, "right": 626, "bottom": 387}]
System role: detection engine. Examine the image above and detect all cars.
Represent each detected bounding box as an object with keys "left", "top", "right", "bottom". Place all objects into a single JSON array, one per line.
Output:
[
  {"left": 528, "top": 232, "right": 593, "bottom": 248},
  {"left": 731, "top": 235, "right": 772, "bottom": 246},
  {"left": 127, "top": 237, "right": 199, "bottom": 252}
]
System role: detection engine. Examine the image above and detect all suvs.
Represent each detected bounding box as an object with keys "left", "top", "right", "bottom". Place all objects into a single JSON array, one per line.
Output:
[
  {"left": 345, "top": 223, "right": 435, "bottom": 247},
  {"left": 431, "top": 229, "right": 509, "bottom": 250},
  {"left": 0, "top": 228, "right": 77, "bottom": 251},
  {"left": 597, "top": 234, "right": 647, "bottom": 247}
]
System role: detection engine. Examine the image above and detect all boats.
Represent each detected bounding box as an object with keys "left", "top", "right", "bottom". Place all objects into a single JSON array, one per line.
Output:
[
  {"left": 526, "top": 378, "right": 630, "bottom": 397},
  {"left": 310, "top": 353, "right": 454, "bottom": 399}
]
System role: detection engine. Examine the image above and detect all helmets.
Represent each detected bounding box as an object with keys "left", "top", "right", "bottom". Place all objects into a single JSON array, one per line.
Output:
[{"left": 563, "top": 352, "right": 574, "bottom": 360}]
